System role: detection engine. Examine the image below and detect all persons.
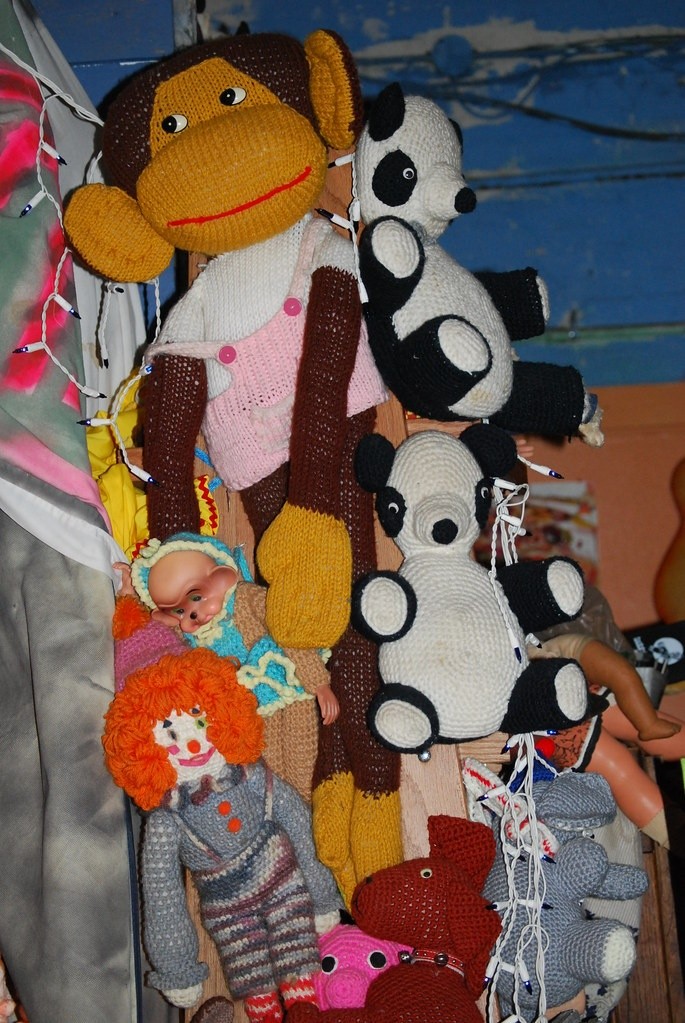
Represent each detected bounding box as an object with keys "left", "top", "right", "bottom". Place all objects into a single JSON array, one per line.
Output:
[{"left": 527, "top": 595, "right": 685, "bottom": 850}]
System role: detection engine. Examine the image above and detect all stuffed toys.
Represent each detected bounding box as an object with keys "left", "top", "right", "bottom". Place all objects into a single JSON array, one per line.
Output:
[
  {"left": 285, "top": 773, "right": 647, "bottom": 1023},
  {"left": 357, "top": 84, "right": 584, "bottom": 449},
  {"left": 101, "top": 650, "right": 344, "bottom": 1023},
  {"left": 0, "top": 0, "right": 135, "bottom": 1022},
  {"left": 64, "top": 27, "right": 399, "bottom": 796},
  {"left": 351, "top": 423, "right": 586, "bottom": 754},
  {"left": 132, "top": 535, "right": 341, "bottom": 799}
]
